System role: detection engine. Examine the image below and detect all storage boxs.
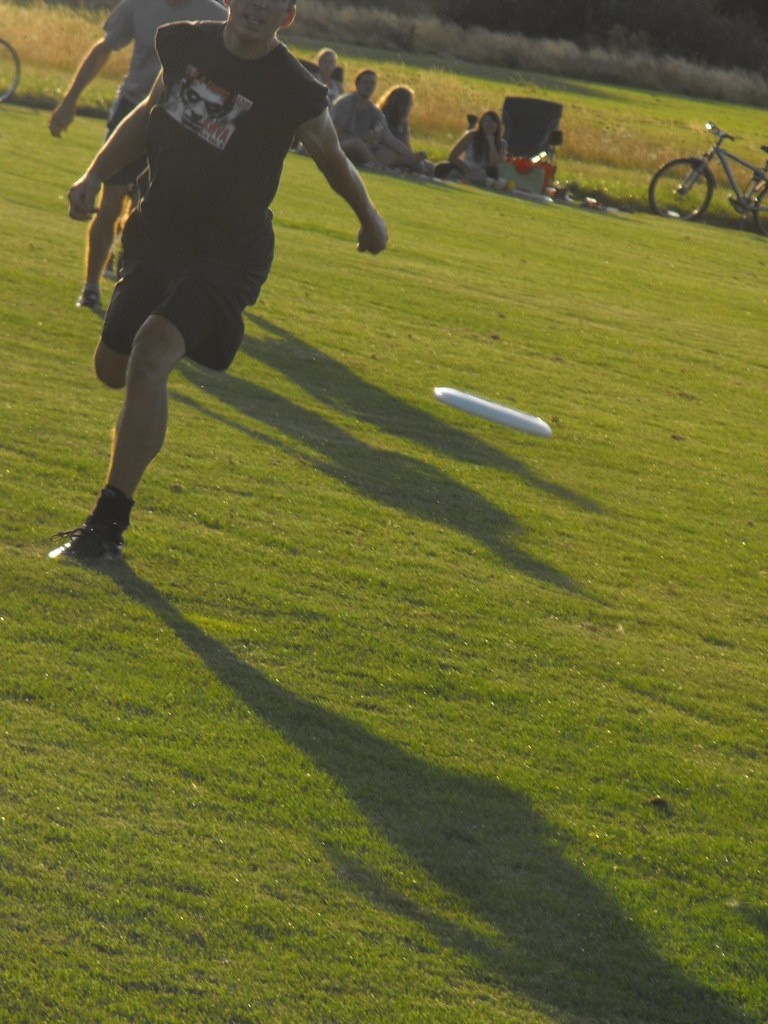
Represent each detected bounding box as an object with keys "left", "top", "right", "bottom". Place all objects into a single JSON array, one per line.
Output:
[{"left": 498, "top": 158, "right": 557, "bottom": 195}]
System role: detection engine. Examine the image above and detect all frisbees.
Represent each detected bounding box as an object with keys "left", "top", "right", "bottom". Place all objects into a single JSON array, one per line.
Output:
[{"left": 429, "top": 383, "right": 555, "bottom": 438}]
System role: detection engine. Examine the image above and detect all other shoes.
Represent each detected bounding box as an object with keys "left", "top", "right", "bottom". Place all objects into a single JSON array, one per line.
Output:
[{"left": 103, "top": 267, "right": 115, "bottom": 279}]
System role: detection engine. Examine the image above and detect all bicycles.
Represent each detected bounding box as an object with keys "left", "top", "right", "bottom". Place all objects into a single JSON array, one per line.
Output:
[
  {"left": 648, "top": 118, "right": 768, "bottom": 238},
  {"left": 0, "top": 38, "right": 21, "bottom": 104}
]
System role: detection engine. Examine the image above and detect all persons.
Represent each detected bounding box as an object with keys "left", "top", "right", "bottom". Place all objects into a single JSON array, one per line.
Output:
[
  {"left": 48, "top": 0, "right": 228, "bottom": 310},
  {"left": 290, "top": 48, "right": 508, "bottom": 190},
  {"left": 48, "top": 0, "right": 387, "bottom": 564}
]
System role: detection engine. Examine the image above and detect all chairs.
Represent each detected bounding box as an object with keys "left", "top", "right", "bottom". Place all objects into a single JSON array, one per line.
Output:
[{"left": 467, "top": 96, "right": 563, "bottom": 156}]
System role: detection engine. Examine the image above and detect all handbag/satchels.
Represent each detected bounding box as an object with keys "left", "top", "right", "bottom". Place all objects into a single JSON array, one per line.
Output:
[{"left": 434, "top": 163, "right": 454, "bottom": 178}]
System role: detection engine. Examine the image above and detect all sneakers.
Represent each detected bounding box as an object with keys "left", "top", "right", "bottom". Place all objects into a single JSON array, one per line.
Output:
[
  {"left": 76, "top": 288, "right": 101, "bottom": 313},
  {"left": 48, "top": 516, "right": 123, "bottom": 571}
]
